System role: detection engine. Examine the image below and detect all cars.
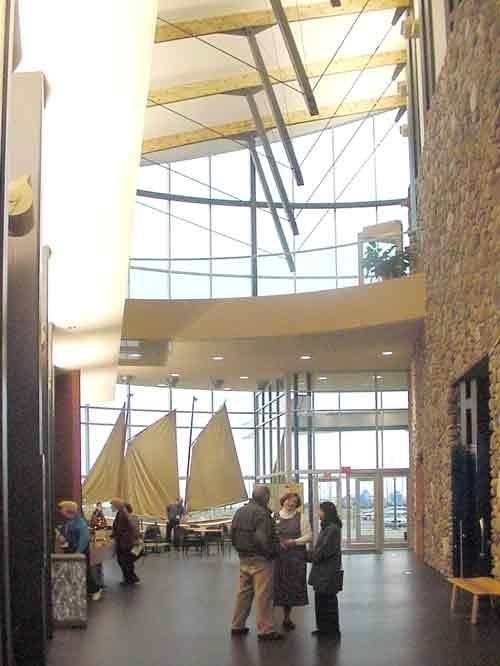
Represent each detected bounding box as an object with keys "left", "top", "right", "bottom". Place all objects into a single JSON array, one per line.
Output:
[{"left": 361, "top": 504, "right": 408, "bottom": 526}]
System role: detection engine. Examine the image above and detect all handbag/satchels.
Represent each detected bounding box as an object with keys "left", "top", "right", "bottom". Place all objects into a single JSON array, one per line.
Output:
[{"left": 336, "top": 570, "right": 344, "bottom": 591}]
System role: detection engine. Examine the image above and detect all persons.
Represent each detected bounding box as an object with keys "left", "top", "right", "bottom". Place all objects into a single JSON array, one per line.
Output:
[
  {"left": 166, "top": 496, "right": 186, "bottom": 542},
  {"left": 126, "top": 504, "right": 139, "bottom": 526},
  {"left": 57, "top": 500, "right": 101, "bottom": 601},
  {"left": 306, "top": 502, "right": 342, "bottom": 639},
  {"left": 231, "top": 486, "right": 281, "bottom": 640},
  {"left": 110, "top": 498, "right": 142, "bottom": 585},
  {"left": 269, "top": 493, "right": 314, "bottom": 630},
  {"left": 92, "top": 503, "right": 106, "bottom": 527}
]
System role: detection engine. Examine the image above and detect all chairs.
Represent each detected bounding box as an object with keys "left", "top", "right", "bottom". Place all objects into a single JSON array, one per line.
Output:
[{"left": 143, "top": 525, "right": 224, "bottom": 552}]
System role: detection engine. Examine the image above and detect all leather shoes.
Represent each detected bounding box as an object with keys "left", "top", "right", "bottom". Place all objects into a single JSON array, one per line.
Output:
[
  {"left": 284, "top": 622, "right": 296, "bottom": 629},
  {"left": 312, "top": 630, "right": 326, "bottom": 635}
]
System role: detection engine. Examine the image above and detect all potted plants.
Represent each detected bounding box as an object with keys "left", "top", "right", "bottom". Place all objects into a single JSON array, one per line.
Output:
[{"left": 358, "top": 240, "right": 410, "bottom": 284}]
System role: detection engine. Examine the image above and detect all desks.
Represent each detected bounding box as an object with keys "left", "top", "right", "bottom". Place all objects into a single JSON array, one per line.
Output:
[{"left": 446, "top": 576, "right": 500, "bottom": 626}]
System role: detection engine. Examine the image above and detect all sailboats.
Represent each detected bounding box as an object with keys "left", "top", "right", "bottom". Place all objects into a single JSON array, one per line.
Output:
[{"left": 81, "top": 401, "right": 249, "bottom": 541}]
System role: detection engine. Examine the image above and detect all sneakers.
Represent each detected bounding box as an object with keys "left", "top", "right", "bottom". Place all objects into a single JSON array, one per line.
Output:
[
  {"left": 92, "top": 587, "right": 103, "bottom": 601},
  {"left": 256, "top": 632, "right": 281, "bottom": 640},
  {"left": 231, "top": 628, "right": 249, "bottom": 634}
]
